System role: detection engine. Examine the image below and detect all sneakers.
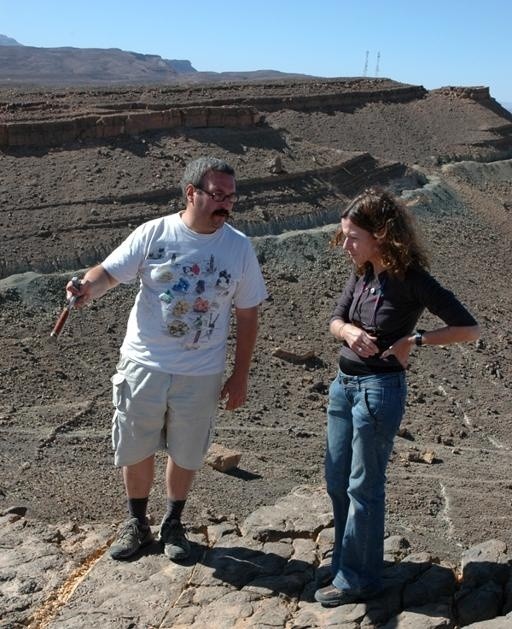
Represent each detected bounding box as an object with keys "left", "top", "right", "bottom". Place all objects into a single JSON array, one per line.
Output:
[
  {"left": 109, "top": 518, "right": 154, "bottom": 560},
  {"left": 159, "top": 512, "right": 192, "bottom": 561},
  {"left": 315, "top": 582, "right": 368, "bottom": 606}
]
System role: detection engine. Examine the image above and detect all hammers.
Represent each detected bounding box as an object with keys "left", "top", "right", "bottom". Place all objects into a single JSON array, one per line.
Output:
[{"left": 50, "top": 276, "right": 83, "bottom": 339}]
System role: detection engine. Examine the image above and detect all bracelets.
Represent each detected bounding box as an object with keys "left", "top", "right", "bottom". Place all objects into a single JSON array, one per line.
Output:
[{"left": 414, "top": 329, "right": 425, "bottom": 348}]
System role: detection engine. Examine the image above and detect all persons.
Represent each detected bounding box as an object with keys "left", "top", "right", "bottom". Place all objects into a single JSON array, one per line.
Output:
[
  {"left": 314, "top": 189, "right": 481, "bottom": 606},
  {"left": 66, "top": 156, "right": 269, "bottom": 561}
]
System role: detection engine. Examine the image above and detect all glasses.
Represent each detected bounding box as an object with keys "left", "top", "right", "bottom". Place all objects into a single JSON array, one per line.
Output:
[{"left": 195, "top": 186, "right": 241, "bottom": 203}]
